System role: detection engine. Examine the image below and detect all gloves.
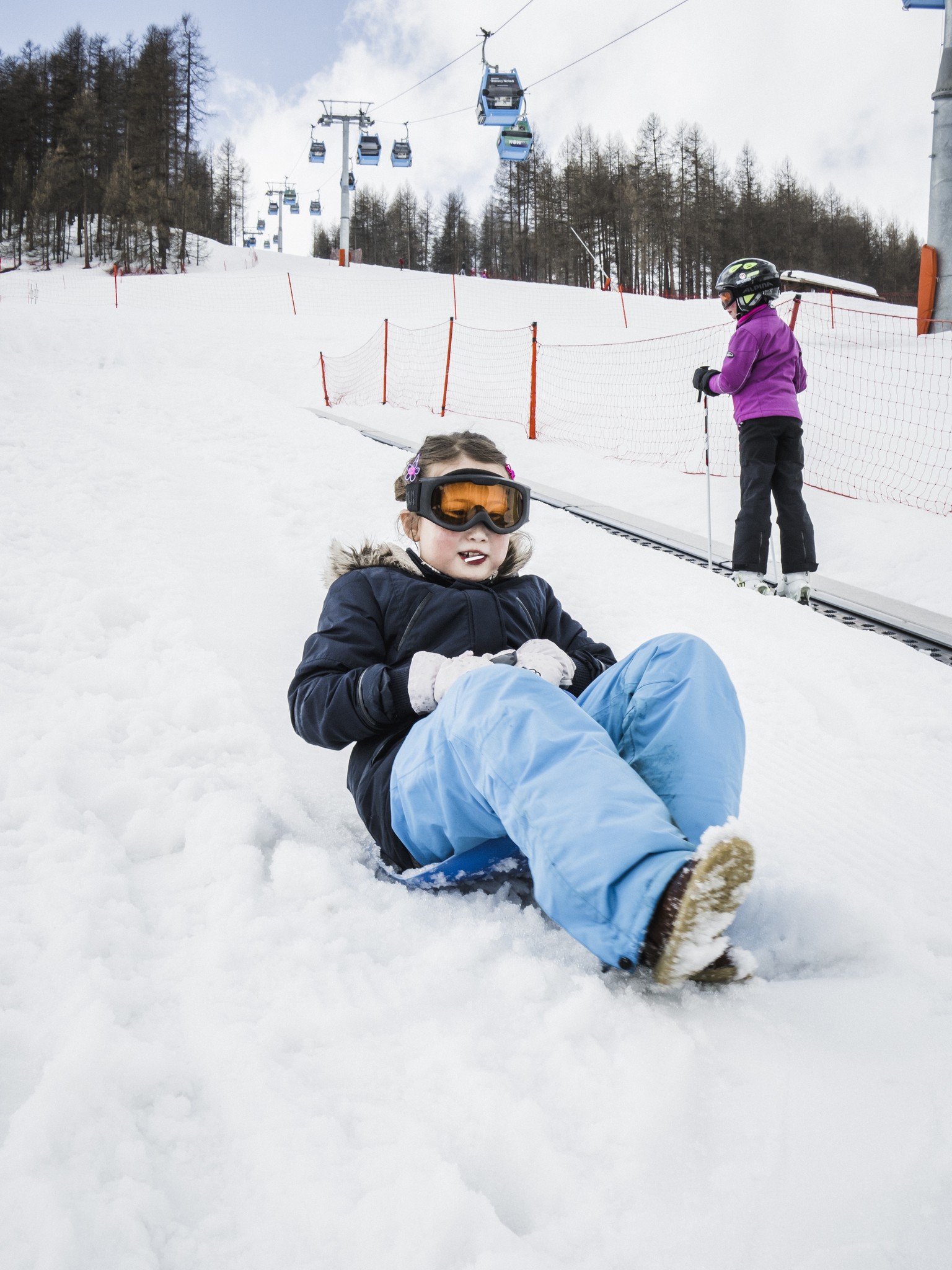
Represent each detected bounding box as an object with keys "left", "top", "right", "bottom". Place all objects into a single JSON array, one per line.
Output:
[
  {"left": 513, "top": 639, "right": 574, "bottom": 690},
  {"left": 408, "top": 649, "right": 517, "bottom": 716},
  {"left": 692, "top": 366, "right": 721, "bottom": 397}
]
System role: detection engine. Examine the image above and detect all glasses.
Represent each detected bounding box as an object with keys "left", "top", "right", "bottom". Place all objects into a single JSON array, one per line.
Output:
[
  {"left": 405, "top": 474, "right": 531, "bottom": 535},
  {"left": 718, "top": 289, "right": 736, "bottom": 310}
]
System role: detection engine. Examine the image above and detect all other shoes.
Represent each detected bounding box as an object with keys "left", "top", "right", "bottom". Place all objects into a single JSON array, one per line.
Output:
[
  {"left": 690, "top": 934, "right": 753, "bottom": 983},
  {"left": 639, "top": 837, "right": 754, "bottom": 987}
]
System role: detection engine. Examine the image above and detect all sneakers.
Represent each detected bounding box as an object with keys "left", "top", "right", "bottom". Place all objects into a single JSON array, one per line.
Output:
[
  {"left": 774, "top": 572, "right": 811, "bottom": 602},
  {"left": 729, "top": 571, "right": 764, "bottom": 590}
]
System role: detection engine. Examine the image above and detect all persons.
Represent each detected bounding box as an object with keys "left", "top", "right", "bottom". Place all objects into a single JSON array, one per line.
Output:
[
  {"left": 287, "top": 430, "right": 761, "bottom": 986},
  {"left": 693, "top": 258, "right": 819, "bottom": 601},
  {"left": 399, "top": 256, "right": 403, "bottom": 271},
  {"left": 460, "top": 262, "right": 465, "bottom": 275}
]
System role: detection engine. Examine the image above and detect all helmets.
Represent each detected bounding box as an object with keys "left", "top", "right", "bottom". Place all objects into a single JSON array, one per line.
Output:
[{"left": 715, "top": 258, "right": 780, "bottom": 311}]
